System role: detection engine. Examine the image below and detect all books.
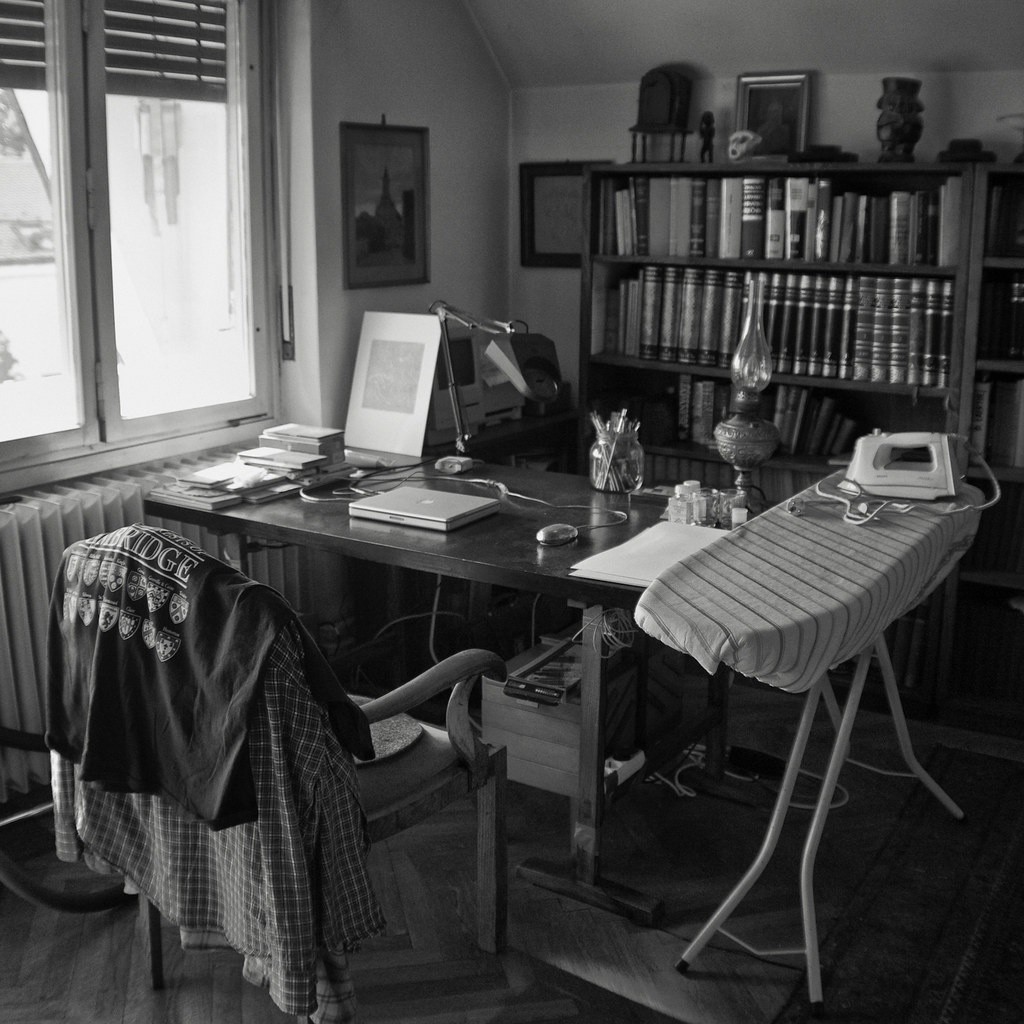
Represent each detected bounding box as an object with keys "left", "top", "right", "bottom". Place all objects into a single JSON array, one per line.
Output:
[
  {"left": 150, "top": 422, "right": 344, "bottom": 510},
  {"left": 937, "top": 139, "right": 996, "bottom": 162},
  {"left": 594, "top": 173, "right": 1024, "bottom": 698},
  {"left": 789, "top": 145, "right": 858, "bottom": 162}
]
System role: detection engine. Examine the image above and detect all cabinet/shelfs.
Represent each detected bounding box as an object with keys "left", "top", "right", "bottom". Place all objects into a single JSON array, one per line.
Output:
[
  {"left": 583, "top": 162, "right": 1023, "bottom": 727},
  {"left": 423, "top": 408, "right": 581, "bottom": 658}
]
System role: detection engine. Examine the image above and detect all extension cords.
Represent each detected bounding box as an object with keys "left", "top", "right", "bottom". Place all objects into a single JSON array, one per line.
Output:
[{"left": 600, "top": 750, "right": 646, "bottom": 793}]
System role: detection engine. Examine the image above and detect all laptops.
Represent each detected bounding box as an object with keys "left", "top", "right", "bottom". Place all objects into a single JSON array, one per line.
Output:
[{"left": 349, "top": 486, "right": 500, "bottom": 532}]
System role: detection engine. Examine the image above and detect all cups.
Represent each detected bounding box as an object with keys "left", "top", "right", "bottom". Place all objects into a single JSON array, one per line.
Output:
[
  {"left": 692, "top": 488, "right": 719, "bottom": 529},
  {"left": 719, "top": 489, "right": 747, "bottom": 527}
]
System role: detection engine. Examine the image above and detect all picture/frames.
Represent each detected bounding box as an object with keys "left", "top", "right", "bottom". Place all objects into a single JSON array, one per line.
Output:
[
  {"left": 518, "top": 161, "right": 613, "bottom": 268},
  {"left": 340, "top": 121, "right": 430, "bottom": 290}
]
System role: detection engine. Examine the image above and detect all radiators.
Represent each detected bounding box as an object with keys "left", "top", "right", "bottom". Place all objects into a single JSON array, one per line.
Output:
[{"left": 1, "top": 453, "right": 301, "bottom": 804}]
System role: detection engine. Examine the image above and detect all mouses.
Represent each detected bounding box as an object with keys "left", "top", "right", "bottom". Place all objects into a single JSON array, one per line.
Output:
[{"left": 535, "top": 523, "right": 578, "bottom": 545}]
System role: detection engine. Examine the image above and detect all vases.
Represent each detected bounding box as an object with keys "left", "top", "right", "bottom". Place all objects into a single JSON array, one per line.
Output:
[{"left": 735, "top": 73, "right": 813, "bottom": 161}]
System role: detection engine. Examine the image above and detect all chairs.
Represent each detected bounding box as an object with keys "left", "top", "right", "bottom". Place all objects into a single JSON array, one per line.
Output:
[{"left": 45, "top": 524, "right": 508, "bottom": 1024}]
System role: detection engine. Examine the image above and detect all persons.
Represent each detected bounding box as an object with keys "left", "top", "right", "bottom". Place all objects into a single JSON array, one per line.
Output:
[{"left": 700, "top": 112, "right": 715, "bottom": 162}]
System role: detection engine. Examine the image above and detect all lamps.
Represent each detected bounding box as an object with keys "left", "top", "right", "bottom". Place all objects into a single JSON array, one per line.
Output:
[
  {"left": 428, "top": 299, "right": 562, "bottom": 456},
  {"left": 714, "top": 281, "right": 780, "bottom": 514}
]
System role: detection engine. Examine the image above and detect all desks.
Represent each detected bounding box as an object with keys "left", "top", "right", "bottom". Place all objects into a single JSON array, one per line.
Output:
[
  {"left": 144, "top": 456, "right": 780, "bottom": 927},
  {"left": 634, "top": 468, "right": 986, "bottom": 1003}
]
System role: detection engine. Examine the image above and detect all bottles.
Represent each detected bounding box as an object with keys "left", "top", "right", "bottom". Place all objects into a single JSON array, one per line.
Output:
[
  {"left": 668, "top": 484, "right": 695, "bottom": 527},
  {"left": 589, "top": 433, "right": 644, "bottom": 493},
  {"left": 683, "top": 481, "right": 707, "bottom": 522}
]
